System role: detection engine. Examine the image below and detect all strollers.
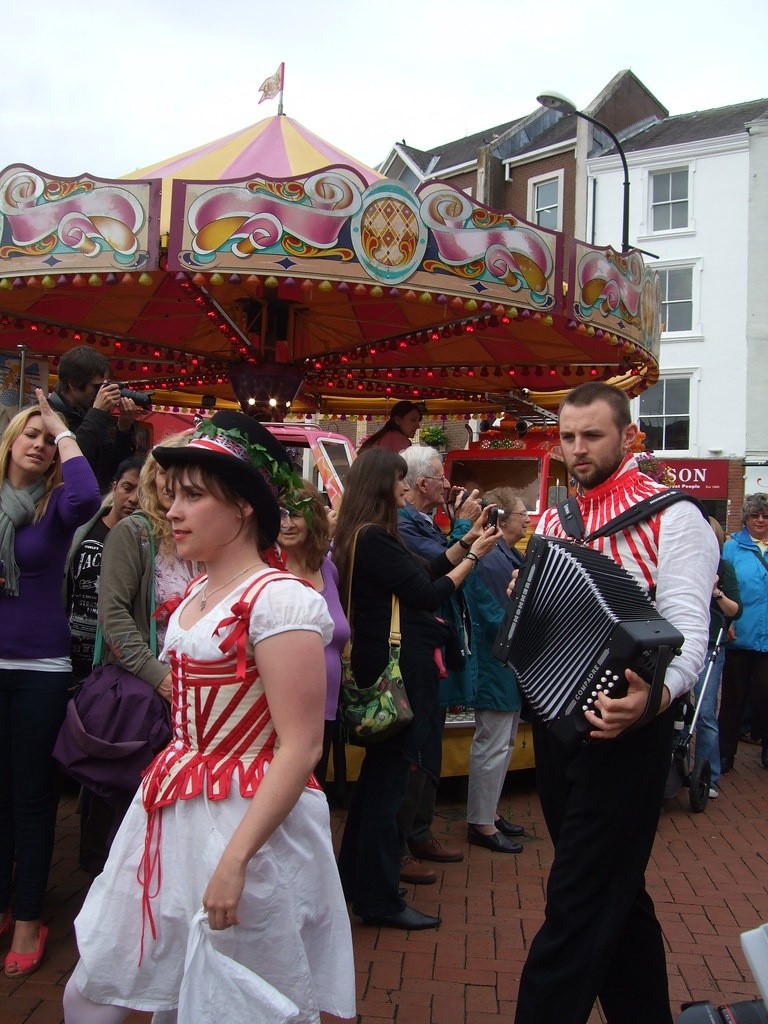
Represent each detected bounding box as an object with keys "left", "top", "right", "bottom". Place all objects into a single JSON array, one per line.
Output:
[{"left": 661, "top": 605, "right": 727, "bottom": 813}]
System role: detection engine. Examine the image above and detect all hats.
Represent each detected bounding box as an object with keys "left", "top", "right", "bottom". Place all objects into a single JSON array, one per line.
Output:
[{"left": 151, "top": 409, "right": 293, "bottom": 552}]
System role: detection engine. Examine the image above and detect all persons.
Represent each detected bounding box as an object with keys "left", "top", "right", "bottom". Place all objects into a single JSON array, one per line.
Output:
[
  {"left": 507, "top": 384, "right": 718, "bottom": 1024},
  {"left": 62, "top": 405, "right": 356, "bottom": 1024},
  {"left": 0, "top": 347, "right": 768, "bottom": 976}
]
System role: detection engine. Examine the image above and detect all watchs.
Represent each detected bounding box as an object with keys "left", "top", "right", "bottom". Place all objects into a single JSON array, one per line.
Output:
[
  {"left": 54, "top": 431, "right": 77, "bottom": 446},
  {"left": 713, "top": 591, "right": 724, "bottom": 601}
]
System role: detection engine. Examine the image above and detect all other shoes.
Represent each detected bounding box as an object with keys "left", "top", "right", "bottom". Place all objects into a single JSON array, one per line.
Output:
[
  {"left": 720, "top": 756, "right": 735, "bottom": 774},
  {"left": 79, "top": 855, "right": 106, "bottom": 871},
  {"left": 761, "top": 739, "right": 768, "bottom": 768},
  {"left": 740, "top": 730, "right": 761, "bottom": 745},
  {"left": 708, "top": 788, "right": 719, "bottom": 798}
]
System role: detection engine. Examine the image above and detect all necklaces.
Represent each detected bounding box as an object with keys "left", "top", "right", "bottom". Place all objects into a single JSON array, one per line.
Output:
[{"left": 200, "top": 563, "right": 269, "bottom": 610}]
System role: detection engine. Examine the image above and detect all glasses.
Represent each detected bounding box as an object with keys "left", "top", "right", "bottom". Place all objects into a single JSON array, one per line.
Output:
[
  {"left": 425, "top": 475, "right": 445, "bottom": 482},
  {"left": 751, "top": 513, "right": 768, "bottom": 519}
]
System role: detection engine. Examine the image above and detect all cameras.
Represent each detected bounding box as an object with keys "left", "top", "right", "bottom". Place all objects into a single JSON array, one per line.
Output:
[
  {"left": 489, "top": 507, "right": 504, "bottom": 536},
  {"left": 104, "top": 382, "right": 155, "bottom": 411},
  {"left": 455, "top": 492, "right": 488, "bottom": 510}
]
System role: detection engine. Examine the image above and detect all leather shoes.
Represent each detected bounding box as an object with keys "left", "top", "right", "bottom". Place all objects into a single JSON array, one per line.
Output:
[
  {"left": 363, "top": 905, "right": 441, "bottom": 930},
  {"left": 408, "top": 837, "right": 465, "bottom": 862},
  {"left": 468, "top": 824, "right": 523, "bottom": 853},
  {"left": 398, "top": 887, "right": 409, "bottom": 896},
  {"left": 398, "top": 853, "right": 438, "bottom": 884},
  {"left": 495, "top": 815, "right": 524, "bottom": 835}
]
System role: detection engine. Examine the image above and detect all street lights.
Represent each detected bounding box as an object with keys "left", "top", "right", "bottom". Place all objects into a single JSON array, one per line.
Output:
[{"left": 537, "top": 88, "right": 631, "bottom": 254}]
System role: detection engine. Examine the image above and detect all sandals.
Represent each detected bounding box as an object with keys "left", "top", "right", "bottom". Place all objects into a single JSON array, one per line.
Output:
[
  {"left": 4, "top": 922, "right": 49, "bottom": 979},
  {"left": 0, "top": 910, "right": 16, "bottom": 939}
]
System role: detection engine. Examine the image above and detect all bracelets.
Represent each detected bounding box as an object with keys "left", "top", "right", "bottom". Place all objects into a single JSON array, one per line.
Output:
[
  {"left": 464, "top": 552, "right": 478, "bottom": 565},
  {"left": 462, "top": 555, "right": 475, "bottom": 572}
]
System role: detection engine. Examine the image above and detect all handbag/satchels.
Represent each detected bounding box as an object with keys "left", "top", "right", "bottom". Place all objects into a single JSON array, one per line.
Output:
[
  {"left": 335, "top": 641, "right": 414, "bottom": 745},
  {"left": 53, "top": 663, "right": 174, "bottom": 804}
]
